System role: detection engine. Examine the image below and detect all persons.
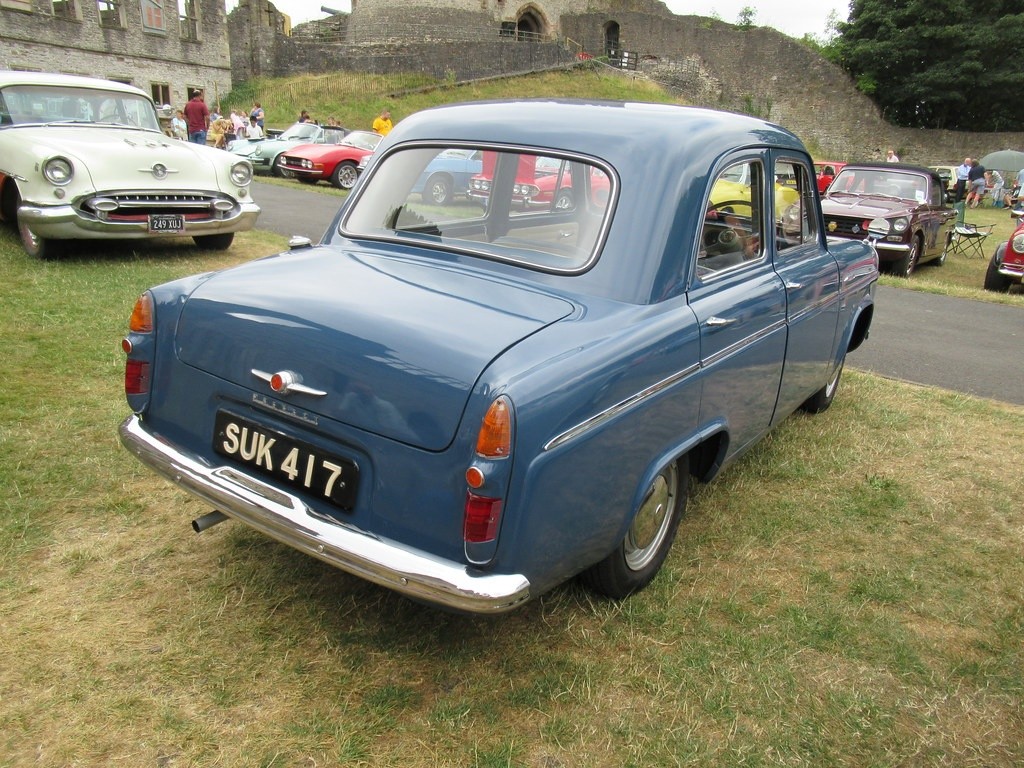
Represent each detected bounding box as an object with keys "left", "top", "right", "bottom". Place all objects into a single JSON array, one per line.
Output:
[
  {"left": 184, "top": 89, "right": 210, "bottom": 145},
  {"left": 299, "top": 110, "right": 314, "bottom": 124},
  {"left": 955, "top": 158, "right": 972, "bottom": 202},
  {"left": 372, "top": 108, "right": 394, "bottom": 136},
  {"left": 964, "top": 159, "right": 986, "bottom": 208},
  {"left": 250, "top": 102, "right": 264, "bottom": 130},
  {"left": 984, "top": 170, "right": 1004, "bottom": 207},
  {"left": 171, "top": 110, "right": 189, "bottom": 141},
  {"left": 887, "top": 149, "right": 899, "bottom": 162},
  {"left": 328, "top": 117, "right": 340, "bottom": 127},
  {"left": 1005, "top": 169, "right": 1024, "bottom": 225},
  {"left": 210, "top": 109, "right": 263, "bottom": 150}
]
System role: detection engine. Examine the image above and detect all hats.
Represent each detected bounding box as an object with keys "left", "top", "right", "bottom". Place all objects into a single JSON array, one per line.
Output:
[{"left": 824, "top": 166, "right": 835, "bottom": 175}]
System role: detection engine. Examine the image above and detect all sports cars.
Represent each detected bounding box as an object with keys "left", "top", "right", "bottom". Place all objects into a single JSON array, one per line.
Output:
[
  {"left": 276, "top": 129, "right": 384, "bottom": 191},
  {"left": 225, "top": 121, "right": 351, "bottom": 178}
]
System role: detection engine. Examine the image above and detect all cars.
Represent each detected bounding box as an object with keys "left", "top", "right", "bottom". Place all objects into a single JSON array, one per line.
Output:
[
  {"left": 0, "top": 68, "right": 262, "bottom": 260},
  {"left": 115, "top": 96, "right": 881, "bottom": 617},
  {"left": 467, "top": 148, "right": 612, "bottom": 212},
  {"left": 983, "top": 209, "right": 1024, "bottom": 294},
  {"left": 781, "top": 161, "right": 959, "bottom": 280},
  {"left": 356, "top": 144, "right": 482, "bottom": 206},
  {"left": 777, "top": 160, "right": 971, "bottom": 203},
  {"left": 709, "top": 163, "right": 800, "bottom": 236}
]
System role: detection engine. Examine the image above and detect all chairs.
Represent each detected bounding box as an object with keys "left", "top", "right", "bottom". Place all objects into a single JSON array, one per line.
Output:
[{"left": 948, "top": 200, "right": 996, "bottom": 259}]
